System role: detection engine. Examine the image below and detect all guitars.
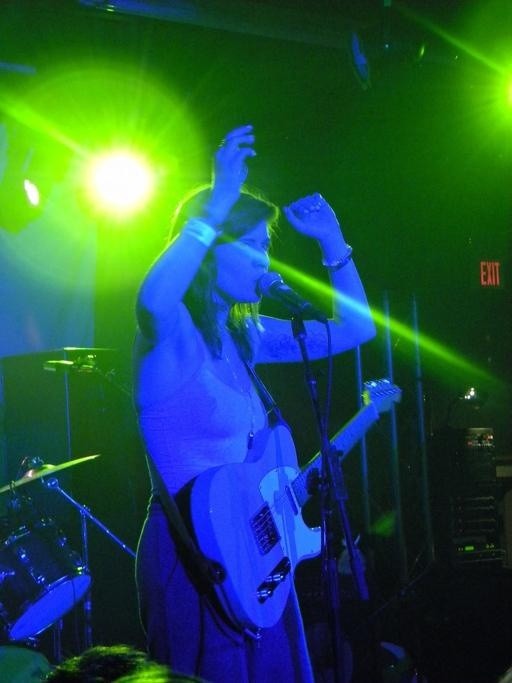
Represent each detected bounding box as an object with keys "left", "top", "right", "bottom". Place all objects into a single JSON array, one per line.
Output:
[{"left": 191, "top": 377, "right": 402, "bottom": 633}]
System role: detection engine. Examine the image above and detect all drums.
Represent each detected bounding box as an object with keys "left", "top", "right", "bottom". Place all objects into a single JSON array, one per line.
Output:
[{"left": 0, "top": 517, "right": 93, "bottom": 642}]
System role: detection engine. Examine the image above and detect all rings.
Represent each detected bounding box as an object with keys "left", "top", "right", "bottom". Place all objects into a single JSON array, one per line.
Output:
[{"left": 217, "top": 146, "right": 226, "bottom": 153}]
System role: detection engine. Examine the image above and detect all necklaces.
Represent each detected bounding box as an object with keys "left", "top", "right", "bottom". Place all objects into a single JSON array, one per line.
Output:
[{"left": 217, "top": 340, "right": 257, "bottom": 446}]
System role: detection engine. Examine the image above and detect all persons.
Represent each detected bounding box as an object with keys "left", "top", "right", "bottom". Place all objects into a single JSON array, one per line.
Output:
[{"left": 135, "top": 124, "right": 379, "bottom": 680}]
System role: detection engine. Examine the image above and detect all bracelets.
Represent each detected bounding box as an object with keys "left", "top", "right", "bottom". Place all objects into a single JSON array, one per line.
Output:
[
  {"left": 322, "top": 244, "right": 355, "bottom": 267},
  {"left": 182, "top": 217, "right": 216, "bottom": 248}
]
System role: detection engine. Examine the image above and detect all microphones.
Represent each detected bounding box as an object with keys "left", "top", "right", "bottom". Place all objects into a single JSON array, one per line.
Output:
[
  {"left": 21, "top": 456, "right": 58, "bottom": 488},
  {"left": 258, "top": 273, "right": 328, "bottom": 325},
  {"left": 43, "top": 360, "right": 94, "bottom": 374}
]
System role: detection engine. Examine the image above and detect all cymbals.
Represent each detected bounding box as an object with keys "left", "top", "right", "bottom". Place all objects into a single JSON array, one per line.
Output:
[{"left": 0, "top": 455, "right": 100, "bottom": 491}]
